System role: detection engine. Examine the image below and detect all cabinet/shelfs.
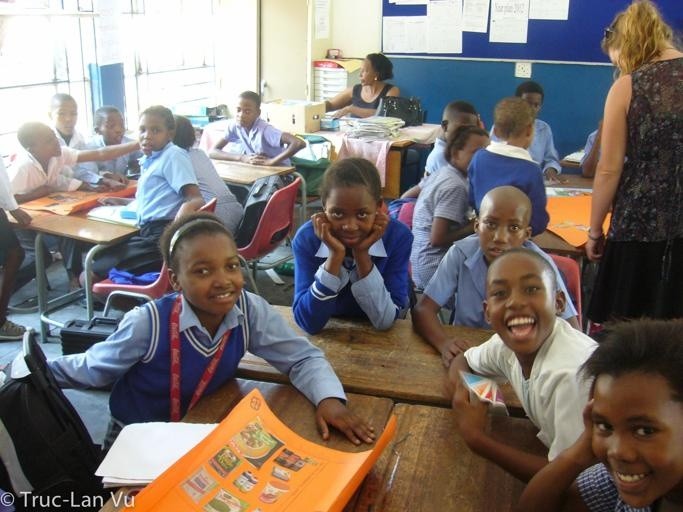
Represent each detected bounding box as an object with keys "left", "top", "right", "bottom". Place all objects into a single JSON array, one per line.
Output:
[{"left": 313, "top": 65, "right": 362, "bottom": 103}]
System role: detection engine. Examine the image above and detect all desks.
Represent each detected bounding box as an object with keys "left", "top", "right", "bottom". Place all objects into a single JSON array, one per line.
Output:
[{"left": 1, "top": 109, "right": 616, "bottom": 512}]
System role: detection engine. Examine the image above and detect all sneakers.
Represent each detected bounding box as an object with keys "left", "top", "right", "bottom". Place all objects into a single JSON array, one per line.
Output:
[
  {"left": 1, "top": 319, "right": 36, "bottom": 341},
  {"left": 69, "top": 287, "right": 102, "bottom": 309}
]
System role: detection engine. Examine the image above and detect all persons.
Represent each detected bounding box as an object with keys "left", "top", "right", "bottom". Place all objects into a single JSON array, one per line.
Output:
[{"left": 0, "top": 1, "right": 682, "bottom": 512}]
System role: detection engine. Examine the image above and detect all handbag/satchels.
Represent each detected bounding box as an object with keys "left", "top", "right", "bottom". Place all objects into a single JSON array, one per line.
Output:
[
  {"left": 235, "top": 175, "right": 291, "bottom": 248},
  {"left": 0, "top": 328, "right": 111, "bottom": 511},
  {"left": 375, "top": 96, "right": 423, "bottom": 127}
]
